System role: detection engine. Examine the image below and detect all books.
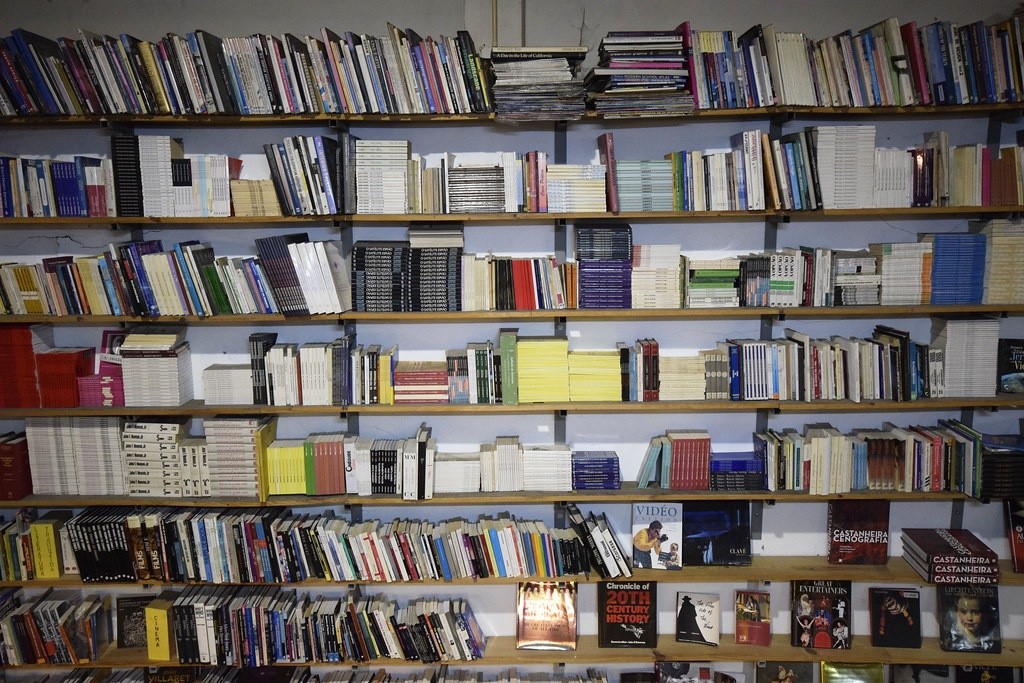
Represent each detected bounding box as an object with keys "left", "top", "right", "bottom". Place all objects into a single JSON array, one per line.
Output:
[{"left": 1, "top": 13, "right": 1024, "bottom": 683}]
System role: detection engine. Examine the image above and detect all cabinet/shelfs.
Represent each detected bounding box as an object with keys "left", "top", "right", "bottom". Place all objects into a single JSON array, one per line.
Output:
[{"left": 0, "top": 102, "right": 1024, "bottom": 683}]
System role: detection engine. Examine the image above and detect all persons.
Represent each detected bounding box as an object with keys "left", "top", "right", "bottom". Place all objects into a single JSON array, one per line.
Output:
[
  {"left": 777, "top": 665, "right": 785, "bottom": 680},
  {"left": 815, "top": 609, "right": 829, "bottom": 630},
  {"left": 797, "top": 614, "right": 816, "bottom": 645},
  {"left": 745, "top": 595, "right": 760, "bottom": 620},
  {"left": 833, "top": 597, "right": 846, "bottom": 617},
  {"left": 801, "top": 594, "right": 813, "bottom": 616},
  {"left": 951, "top": 592, "right": 996, "bottom": 647},
  {"left": 830, "top": 620, "right": 848, "bottom": 648},
  {"left": 785, "top": 670, "right": 795, "bottom": 683},
  {"left": 633, "top": 521, "right": 663, "bottom": 568},
  {"left": 665, "top": 543, "right": 679, "bottom": 568}
]
